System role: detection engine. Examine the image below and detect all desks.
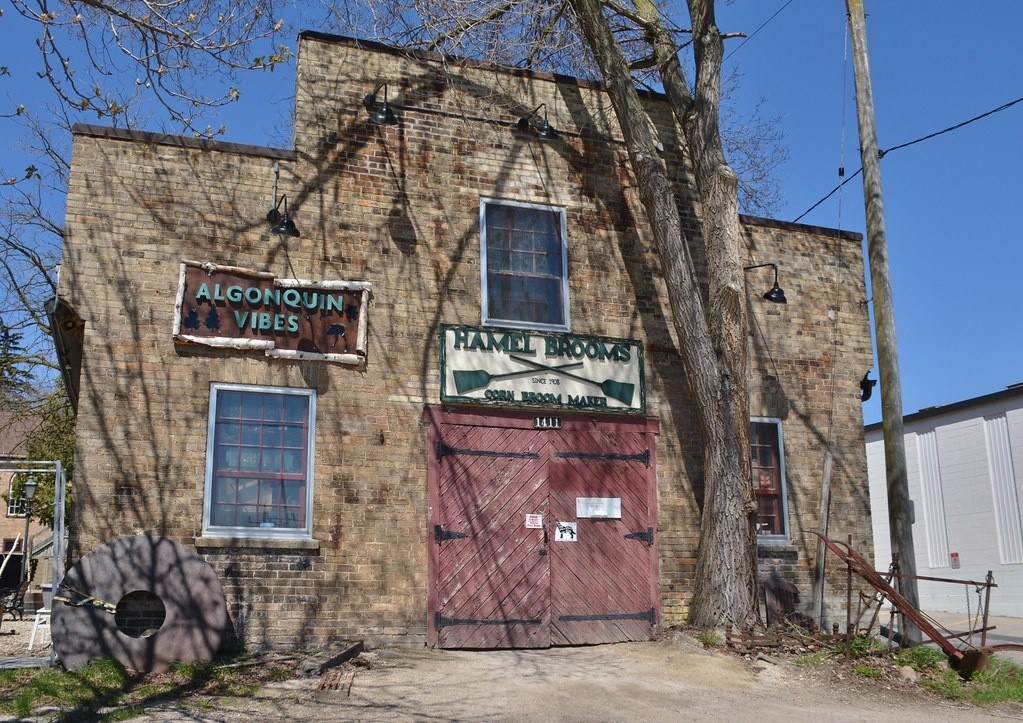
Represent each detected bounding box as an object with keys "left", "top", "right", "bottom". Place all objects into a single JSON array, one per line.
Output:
[{"left": 28, "top": 607, "right": 52, "bottom": 650}]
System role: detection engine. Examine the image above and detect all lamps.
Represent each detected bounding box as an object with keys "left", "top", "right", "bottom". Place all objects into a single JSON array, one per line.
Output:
[
  {"left": 363, "top": 82, "right": 400, "bottom": 125},
  {"left": 518, "top": 103, "right": 558, "bottom": 141},
  {"left": 742, "top": 263, "right": 788, "bottom": 304},
  {"left": 267, "top": 162, "right": 300, "bottom": 239}
]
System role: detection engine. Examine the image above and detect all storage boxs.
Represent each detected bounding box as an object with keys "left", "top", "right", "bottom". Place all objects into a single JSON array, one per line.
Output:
[{"left": 230, "top": 483, "right": 274, "bottom": 512}]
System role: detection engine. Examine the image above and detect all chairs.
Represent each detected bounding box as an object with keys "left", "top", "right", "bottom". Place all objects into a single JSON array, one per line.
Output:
[{"left": 0, "top": 579, "right": 30, "bottom": 623}]
